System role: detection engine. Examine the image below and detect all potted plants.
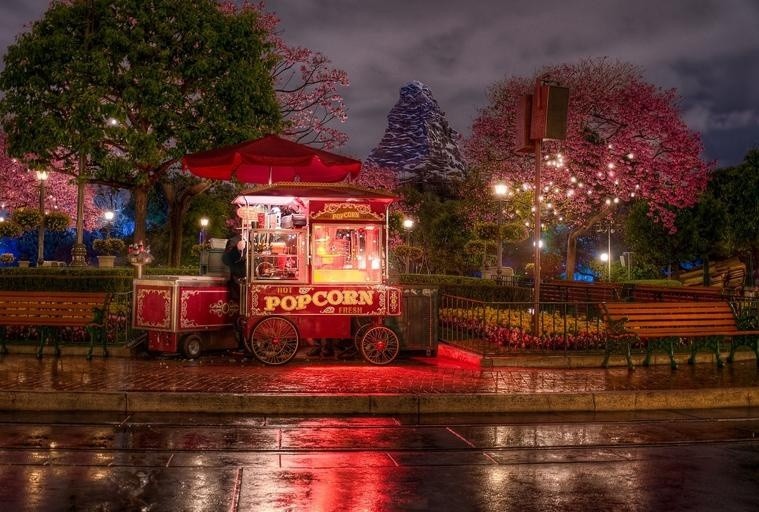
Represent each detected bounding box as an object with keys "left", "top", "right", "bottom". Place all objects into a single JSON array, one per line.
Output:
[{"left": 93, "top": 238, "right": 123, "bottom": 268}]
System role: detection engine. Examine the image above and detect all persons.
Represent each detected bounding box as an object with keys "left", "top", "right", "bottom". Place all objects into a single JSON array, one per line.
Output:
[
  {"left": 223, "top": 217, "right": 247, "bottom": 282},
  {"left": 306, "top": 337, "right": 336, "bottom": 357}
]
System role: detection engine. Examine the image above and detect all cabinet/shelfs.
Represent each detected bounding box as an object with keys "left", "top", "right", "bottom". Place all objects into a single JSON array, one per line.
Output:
[{"left": 249, "top": 226, "right": 306, "bottom": 282}]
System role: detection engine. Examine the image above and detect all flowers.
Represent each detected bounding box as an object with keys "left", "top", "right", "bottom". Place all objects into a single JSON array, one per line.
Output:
[{"left": 126, "top": 241, "right": 155, "bottom": 266}]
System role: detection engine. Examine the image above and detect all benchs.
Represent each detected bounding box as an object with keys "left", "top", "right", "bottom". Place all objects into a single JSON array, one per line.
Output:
[
  {"left": 596, "top": 301, "right": 757, "bottom": 375},
  {"left": 533, "top": 282, "right": 748, "bottom": 312},
  {"left": 0, "top": 291, "right": 114, "bottom": 359}
]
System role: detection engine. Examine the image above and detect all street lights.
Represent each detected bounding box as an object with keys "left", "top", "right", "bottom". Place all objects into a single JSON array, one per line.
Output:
[
  {"left": 495, "top": 183, "right": 507, "bottom": 284},
  {"left": 400, "top": 220, "right": 414, "bottom": 273},
  {"left": 597, "top": 217, "right": 624, "bottom": 283},
  {"left": 35, "top": 171, "right": 49, "bottom": 266}
]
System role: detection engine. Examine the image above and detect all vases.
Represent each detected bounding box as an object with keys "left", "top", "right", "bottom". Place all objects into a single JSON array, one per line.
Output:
[{"left": 18, "top": 260, "right": 32, "bottom": 268}]
